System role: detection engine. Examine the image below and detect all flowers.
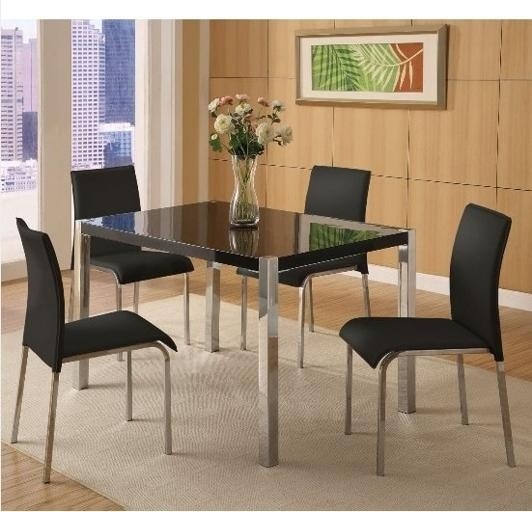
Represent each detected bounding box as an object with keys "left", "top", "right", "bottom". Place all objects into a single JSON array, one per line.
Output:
[{"left": 199, "top": 92, "right": 296, "bottom": 185}]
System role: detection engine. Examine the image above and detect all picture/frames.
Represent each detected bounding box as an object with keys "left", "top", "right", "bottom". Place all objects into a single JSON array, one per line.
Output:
[{"left": 291, "top": 23, "right": 451, "bottom": 113}]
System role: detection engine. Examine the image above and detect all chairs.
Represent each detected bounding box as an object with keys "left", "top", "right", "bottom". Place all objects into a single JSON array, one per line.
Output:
[
  {"left": 234, "top": 165, "right": 378, "bottom": 370},
  {"left": 68, "top": 161, "right": 191, "bottom": 362},
  {"left": 10, "top": 215, "right": 182, "bottom": 486},
  {"left": 335, "top": 201, "right": 520, "bottom": 478}
]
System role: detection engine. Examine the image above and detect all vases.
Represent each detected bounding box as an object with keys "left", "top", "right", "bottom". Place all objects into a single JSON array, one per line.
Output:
[{"left": 226, "top": 156, "right": 264, "bottom": 227}]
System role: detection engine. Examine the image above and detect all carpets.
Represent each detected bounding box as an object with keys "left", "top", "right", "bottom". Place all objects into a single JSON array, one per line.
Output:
[{"left": 2, "top": 291, "right": 532, "bottom": 511}]
